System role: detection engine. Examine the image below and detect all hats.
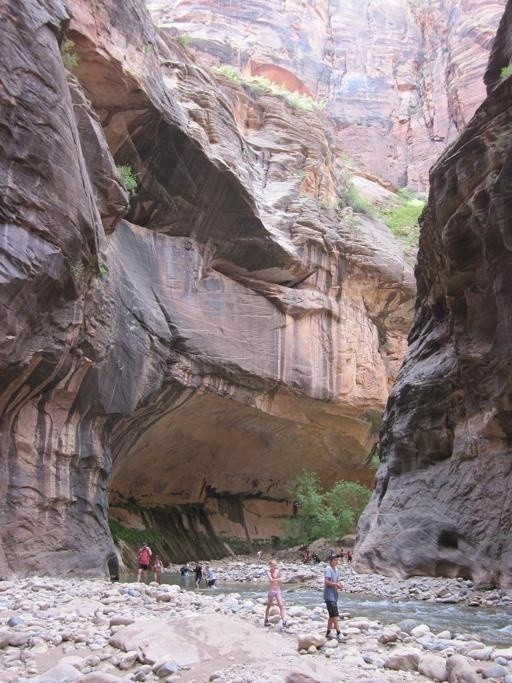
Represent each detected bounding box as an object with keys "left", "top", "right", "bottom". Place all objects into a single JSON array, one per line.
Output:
[{"left": 328, "top": 553, "right": 340, "bottom": 559}]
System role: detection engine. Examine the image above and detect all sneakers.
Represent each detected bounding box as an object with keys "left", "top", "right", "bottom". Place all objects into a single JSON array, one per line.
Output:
[
  {"left": 326, "top": 632, "right": 337, "bottom": 638},
  {"left": 336, "top": 633, "right": 347, "bottom": 642},
  {"left": 282, "top": 621, "right": 290, "bottom": 628},
  {"left": 265, "top": 621, "right": 274, "bottom": 626}
]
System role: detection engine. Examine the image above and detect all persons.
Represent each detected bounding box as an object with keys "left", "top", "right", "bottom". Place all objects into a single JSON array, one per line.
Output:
[
  {"left": 302, "top": 544, "right": 353, "bottom": 563},
  {"left": 180, "top": 560, "right": 217, "bottom": 590},
  {"left": 151, "top": 555, "right": 164, "bottom": 584},
  {"left": 323, "top": 552, "right": 346, "bottom": 643},
  {"left": 263, "top": 558, "right": 291, "bottom": 628},
  {"left": 136, "top": 542, "right": 152, "bottom": 583}
]
